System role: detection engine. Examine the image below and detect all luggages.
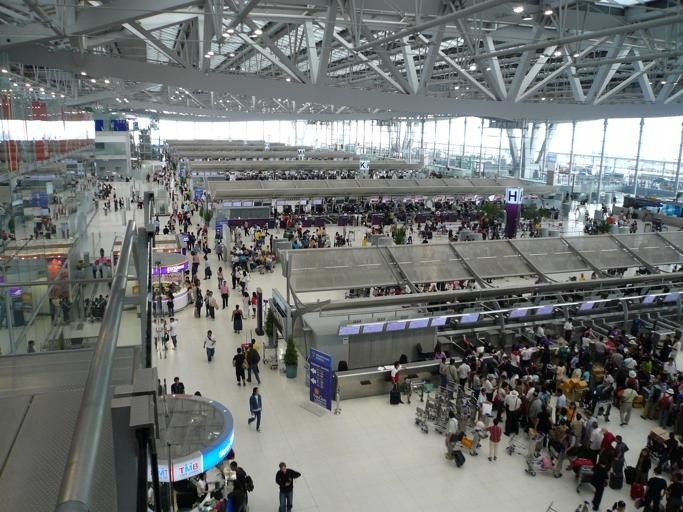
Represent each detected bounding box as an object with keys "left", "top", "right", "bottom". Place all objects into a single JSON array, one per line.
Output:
[
  {"left": 632, "top": 394, "right": 643, "bottom": 408},
  {"left": 630, "top": 481, "right": 644, "bottom": 499},
  {"left": 608, "top": 472, "right": 623, "bottom": 489},
  {"left": 389, "top": 382, "right": 401, "bottom": 405},
  {"left": 451, "top": 443, "right": 465, "bottom": 467},
  {"left": 622, "top": 460, "right": 636, "bottom": 484}
]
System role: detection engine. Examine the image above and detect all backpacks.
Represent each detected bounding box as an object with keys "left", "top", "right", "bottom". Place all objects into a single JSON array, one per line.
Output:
[{"left": 658, "top": 394, "right": 670, "bottom": 411}]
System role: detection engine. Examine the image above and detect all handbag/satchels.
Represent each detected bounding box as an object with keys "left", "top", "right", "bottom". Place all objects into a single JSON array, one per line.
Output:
[
  {"left": 649, "top": 386, "right": 661, "bottom": 404},
  {"left": 566, "top": 446, "right": 594, "bottom": 483},
  {"left": 450, "top": 434, "right": 458, "bottom": 442},
  {"left": 245, "top": 475, "right": 255, "bottom": 493}
]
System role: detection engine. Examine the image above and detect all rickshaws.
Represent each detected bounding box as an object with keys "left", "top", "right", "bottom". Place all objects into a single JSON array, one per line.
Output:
[
  {"left": 644, "top": 435, "right": 669, "bottom": 467},
  {"left": 506, "top": 434, "right": 595, "bottom": 492},
  {"left": 403, "top": 377, "right": 477, "bottom": 432}
]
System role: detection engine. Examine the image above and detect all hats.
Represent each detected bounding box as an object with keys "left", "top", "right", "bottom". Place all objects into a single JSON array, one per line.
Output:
[
  {"left": 628, "top": 370, "right": 637, "bottom": 378},
  {"left": 509, "top": 389, "right": 519, "bottom": 397},
  {"left": 664, "top": 388, "right": 674, "bottom": 395}
]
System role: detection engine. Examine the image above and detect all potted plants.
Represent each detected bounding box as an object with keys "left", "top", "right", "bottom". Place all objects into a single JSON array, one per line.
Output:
[
  {"left": 282, "top": 334, "right": 298, "bottom": 378},
  {"left": 263, "top": 311, "right": 277, "bottom": 347}
]
